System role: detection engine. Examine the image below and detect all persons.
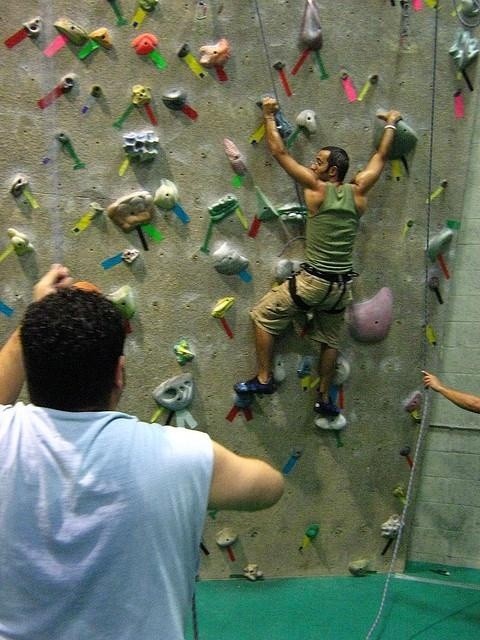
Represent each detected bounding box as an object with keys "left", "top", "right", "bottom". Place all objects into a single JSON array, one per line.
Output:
[
  {"left": 234, "top": 98, "right": 400, "bottom": 411},
  {"left": 1, "top": 263, "right": 287, "bottom": 637},
  {"left": 420, "top": 370, "right": 480, "bottom": 411}
]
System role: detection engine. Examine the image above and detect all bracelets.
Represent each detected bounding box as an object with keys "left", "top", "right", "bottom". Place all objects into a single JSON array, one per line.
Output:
[
  {"left": 264, "top": 116, "right": 276, "bottom": 121},
  {"left": 383, "top": 122, "right": 396, "bottom": 130}
]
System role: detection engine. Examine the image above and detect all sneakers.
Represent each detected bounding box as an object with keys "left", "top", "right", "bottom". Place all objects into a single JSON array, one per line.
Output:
[
  {"left": 233, "top": 376, "right": 273, "bottom": 395},
  {"left": 314, "top": 396, "right": 340, "bottom": 416}
]
